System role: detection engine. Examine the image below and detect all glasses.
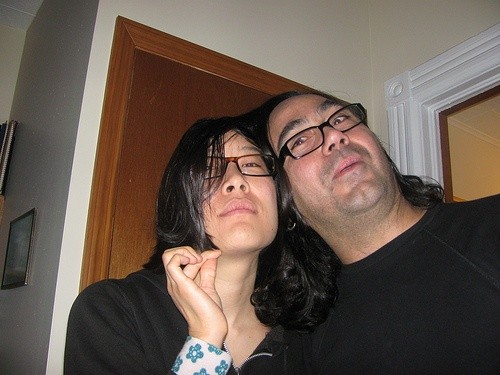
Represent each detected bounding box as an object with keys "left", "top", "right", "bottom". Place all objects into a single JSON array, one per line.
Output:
[
  {"left": 277, "top": 103, "right": 368, "bottom": 169},
  {"left": 203, "top": 154, "right": 277, "bottom": 180}
]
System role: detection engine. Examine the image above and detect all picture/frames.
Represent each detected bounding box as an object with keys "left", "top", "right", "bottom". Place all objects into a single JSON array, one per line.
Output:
[{"left": 1, "top": 207, "right": 38, "bottom": 289}]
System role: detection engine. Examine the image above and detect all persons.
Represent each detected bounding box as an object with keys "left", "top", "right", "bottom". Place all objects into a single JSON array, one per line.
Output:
[
  {"left": 254, "top": 90, "right": 500, "bottom": 375},
  {"left": 64, "top": 117, "right": 307, "bottom": 375}
]
